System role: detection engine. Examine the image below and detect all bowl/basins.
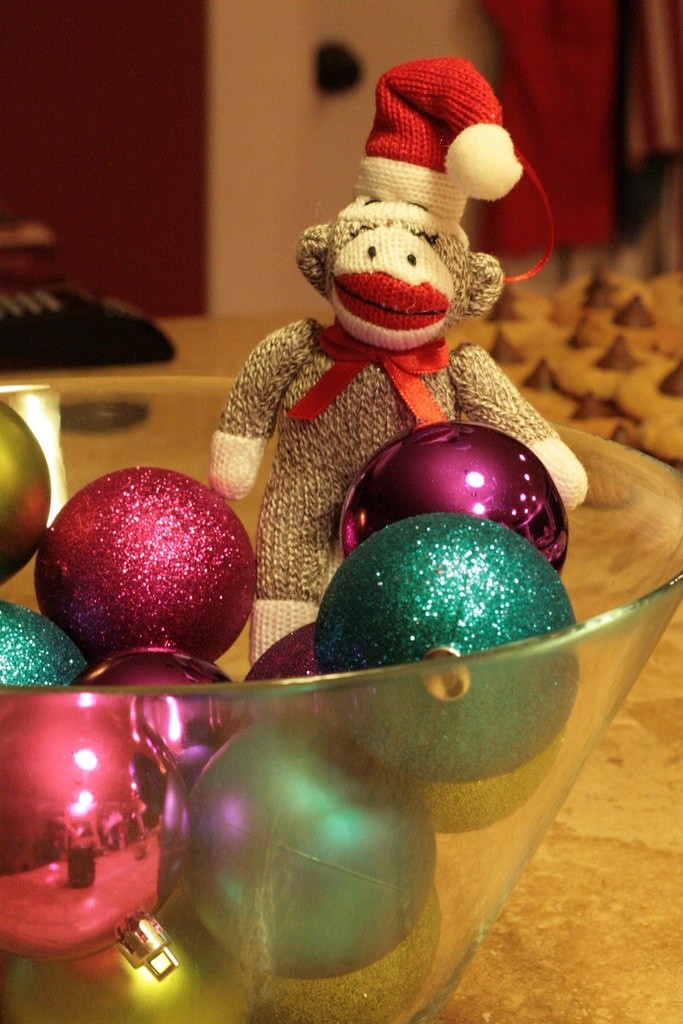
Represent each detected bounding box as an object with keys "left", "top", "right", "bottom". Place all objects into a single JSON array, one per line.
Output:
[{"left": 0, "top": 375, "right": 683, "bottom": 1024}]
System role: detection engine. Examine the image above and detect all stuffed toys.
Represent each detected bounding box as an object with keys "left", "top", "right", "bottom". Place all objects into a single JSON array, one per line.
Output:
[{"left": 205, "top": 57, "right": 587, "bottom": 681}]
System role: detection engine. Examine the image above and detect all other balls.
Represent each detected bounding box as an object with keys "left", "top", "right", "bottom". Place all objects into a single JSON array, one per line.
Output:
[{"left": 0, "top": 399, "right": 580, "bottom": 1024}]
